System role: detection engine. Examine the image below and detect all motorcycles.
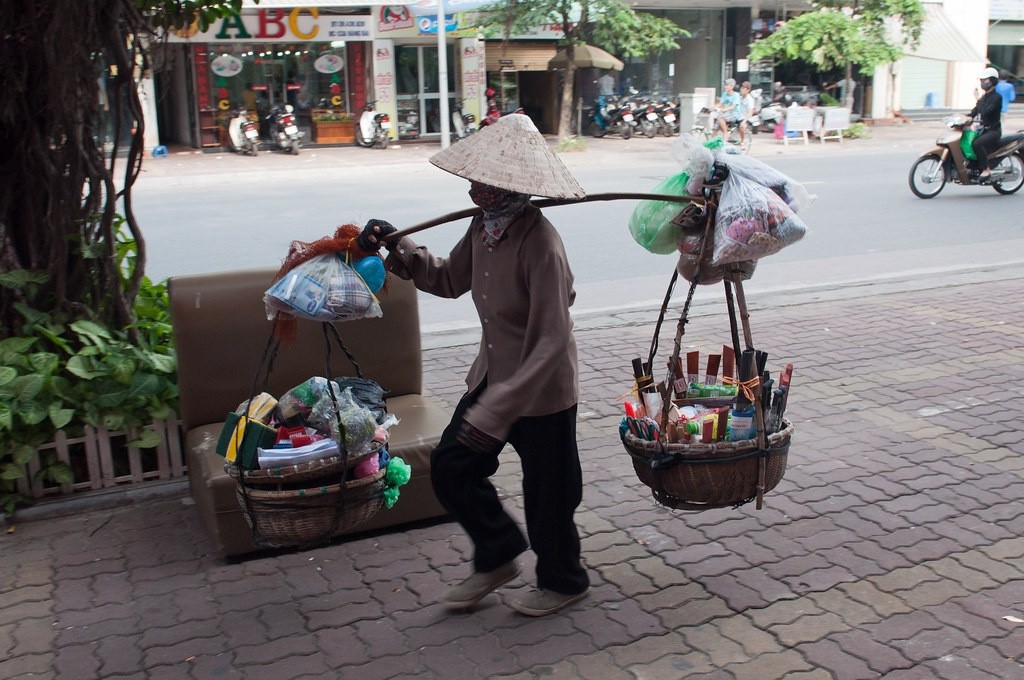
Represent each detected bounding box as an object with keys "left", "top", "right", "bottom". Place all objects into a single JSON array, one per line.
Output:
[
  {"left": 355, "top": 99, "right": 393, "bottom": 150},
  {"left": 746, "top": 81, "right": 829, "bottom": 134},
  {"left": 683, "top": 104, "right": 753, "bottom": 155},
  {"left": 478, "top": 87, "right": 524, "bottom": 131},
  {"left": 908, "top": 112, "right": 1024, "bottom": 200},
  {"left": 588, "top": 84, "right": 682, "bottom": 140},
  {"left": 256, "top": 96, "right": 306, "bottom": 155},
  {"left": 449, "top": 96, "right": 477, "bottom": 141},
  {"left": 222, "top": 103, "right": 259, "bottom": 157}
]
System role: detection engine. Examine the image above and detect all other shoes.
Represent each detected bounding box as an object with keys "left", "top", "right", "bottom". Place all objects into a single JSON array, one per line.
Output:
[
  {"left": 439, "top": 560, "right": 524, "bottom": 608},
  {"left": 504, "top": 586, "right": 592, "bottom": 616},
  {"left": 978, "top": 173, "right": 990, "bottom": 180}
]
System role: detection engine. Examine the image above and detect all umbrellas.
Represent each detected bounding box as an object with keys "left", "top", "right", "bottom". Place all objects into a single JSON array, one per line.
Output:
[{"left": 548, "top": 45, "right": 625, "bottom": 139}]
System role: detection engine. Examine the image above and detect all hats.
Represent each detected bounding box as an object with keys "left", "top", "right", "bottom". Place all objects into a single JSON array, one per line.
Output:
[
  {"left": 428, "top": 113, "right": 586, "bottom": 200},
  {"left": 724, "top": 78, "right": 736, "bottom": 86},
  {"left": 739, "top": 81, "right": 751, "bottom": 89}
]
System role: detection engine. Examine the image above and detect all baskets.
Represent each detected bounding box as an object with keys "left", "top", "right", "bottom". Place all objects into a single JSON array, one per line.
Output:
[
  {"left": 236, "top": 467, "right": 388, "bottom": 548},
  {"left": 618, "top": 398, "right": 794, "bottom": 511}
]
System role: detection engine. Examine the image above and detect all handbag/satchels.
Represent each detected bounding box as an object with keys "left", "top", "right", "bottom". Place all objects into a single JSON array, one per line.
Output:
[
  {"left": 629, "top": 135, "right": 817, "bottom": 266},
  {"left": 960, "top": 130, "right": 980, "bottom": 160},
  {"left": 263, "top": 253, "right": 383, "bottom": 322}
]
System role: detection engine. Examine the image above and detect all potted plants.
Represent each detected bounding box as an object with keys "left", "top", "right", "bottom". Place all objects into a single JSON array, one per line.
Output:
[
  {"left": 216, "top": 114, "right": 259, "bottom": 146},
  {"left": 312, "top": 113, "right": 356, "bottom": 144}
]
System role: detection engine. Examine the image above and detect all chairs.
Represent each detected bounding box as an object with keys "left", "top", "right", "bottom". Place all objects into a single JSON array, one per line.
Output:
[{"left": 781, "top": 106, "right": 851, "bottom": 146}]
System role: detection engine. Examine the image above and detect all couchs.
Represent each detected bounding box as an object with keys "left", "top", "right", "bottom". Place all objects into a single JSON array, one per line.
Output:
[{"left": 169, "top": 265, "right": 455, "bottom": 563}]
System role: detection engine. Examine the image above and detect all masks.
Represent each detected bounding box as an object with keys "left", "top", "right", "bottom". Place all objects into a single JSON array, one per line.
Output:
[{"left": 981, "top": 82, "right": 991, "bottom": 90}]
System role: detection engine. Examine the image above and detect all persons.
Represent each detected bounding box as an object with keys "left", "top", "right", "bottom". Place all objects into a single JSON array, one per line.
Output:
[
  {"left": 596, "top": 72, "right": 616, "bottom": 95},
  {"left": 96, "top": 76, "right": 109, "bottom": 146},
  {"left": 823, "top": 76, "right": 856, "bottom": 112},
  {"left": 356, "top": 111, "right": 592, "bottom": 617},
  {"left": 241, "top": 71, "right": 299, "bottom": 129},
  {"left": 711, "top": 77, "right": 755, "bottom": 146},
  {"left": 966, "top": 68, "right": 1015, "bottom": 182}
]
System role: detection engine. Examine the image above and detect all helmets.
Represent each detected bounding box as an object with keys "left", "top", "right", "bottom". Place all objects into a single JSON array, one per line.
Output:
[{"left": 977, "top": 68, "right": 998, "bottom": 79}]
